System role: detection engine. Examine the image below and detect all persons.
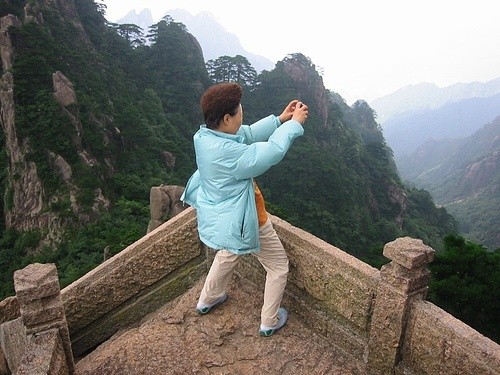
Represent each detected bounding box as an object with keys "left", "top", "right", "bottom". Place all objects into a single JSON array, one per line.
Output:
[{"left": 180, "top": 82, "right": 309, "bottom": 337}]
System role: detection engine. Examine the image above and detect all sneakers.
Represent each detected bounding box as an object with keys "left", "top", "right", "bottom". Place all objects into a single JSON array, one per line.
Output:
[
  {"left": 196, "top": 291, "right": 228, "bottom": 314},
  {"left": 260, "top": 307, "right": 288, "bottom": 336}
]
